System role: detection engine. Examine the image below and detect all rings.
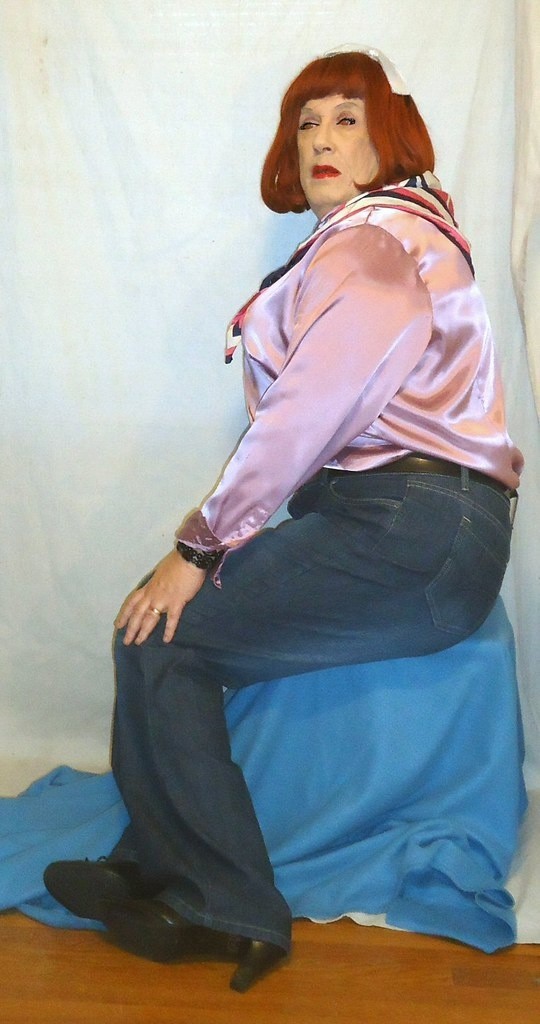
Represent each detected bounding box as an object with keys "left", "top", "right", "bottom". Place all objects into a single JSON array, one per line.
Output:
[{"left": 149, "top": 607, "right": 161, "bottom": 615}]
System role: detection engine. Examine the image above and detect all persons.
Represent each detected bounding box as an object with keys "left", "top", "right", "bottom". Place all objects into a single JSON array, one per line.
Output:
[{"left": 43, "top": 48, "right": 522, "bottom": 993}]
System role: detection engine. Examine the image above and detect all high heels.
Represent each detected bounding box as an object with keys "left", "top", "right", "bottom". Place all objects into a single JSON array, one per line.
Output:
[
  {"left": 94, "top": 898, "right": 287, "bottom": 994},
  {"left": 43, "top": 856, "right": 153, "bottom": 921}
]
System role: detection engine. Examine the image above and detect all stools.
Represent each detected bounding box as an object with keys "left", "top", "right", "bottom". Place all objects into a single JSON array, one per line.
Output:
[{"left": 224, "top": 590, "right": 522, "bottom": 939}]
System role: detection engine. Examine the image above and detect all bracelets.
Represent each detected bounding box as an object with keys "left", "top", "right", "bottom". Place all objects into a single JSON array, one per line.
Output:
[{"left": 177, "top": 540, "right": 224, "bottom": 570}]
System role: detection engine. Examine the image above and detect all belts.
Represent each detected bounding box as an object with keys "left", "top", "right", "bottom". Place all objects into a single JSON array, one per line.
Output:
[{"left": 305, "top": 452, "right": 512, "bottom": 498}]
